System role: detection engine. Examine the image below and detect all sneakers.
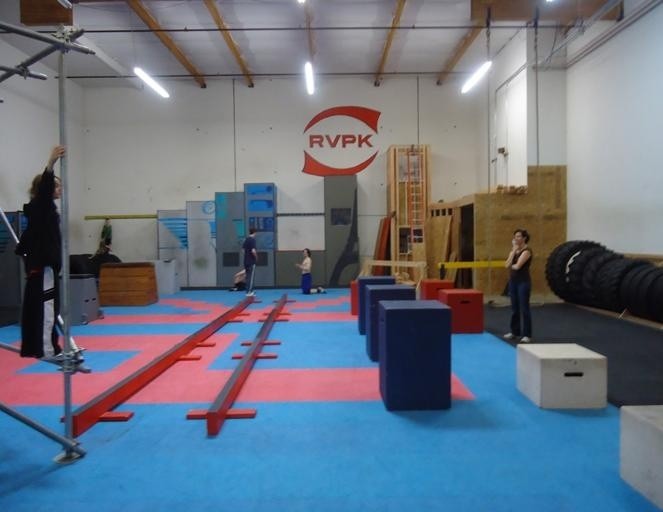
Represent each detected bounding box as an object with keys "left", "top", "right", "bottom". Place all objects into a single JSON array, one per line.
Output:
[
  {"left": 317, "top": 287, "right": 326, "bottom": 293},
  {"left": 246, "top": 289, "right": 256, "bottom": 296},
  {"left": 504, "top": 332, "right": 530, "bottom": 342}
]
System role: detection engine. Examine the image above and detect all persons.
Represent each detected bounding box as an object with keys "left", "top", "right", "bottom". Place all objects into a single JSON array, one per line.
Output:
[
  {"left": 231, "top": 269, "right": 246, "bottom": 291},
  {"left": 243, "top": 228, "right": 259, "bottom": 296},
  {"left": 14, "top": 145, "right": 65, "bottom": 357},
  {"left": 504, "top": 229, "right": 533, "bottom": 343},
  {"left": 295, "top": 248, "right": 327, "bottom": 294}
]
